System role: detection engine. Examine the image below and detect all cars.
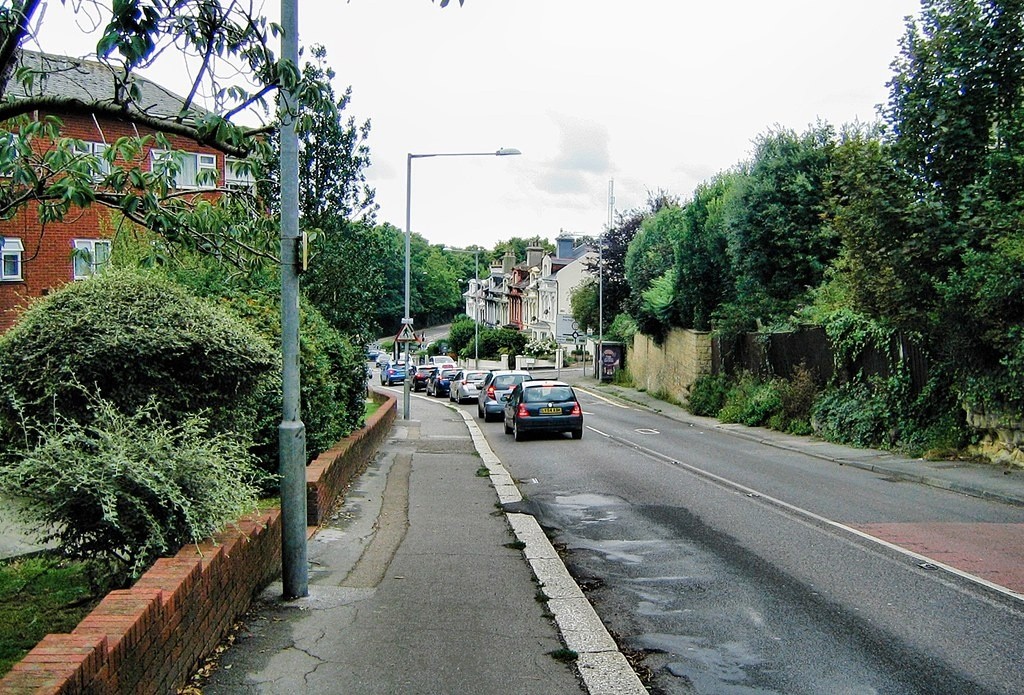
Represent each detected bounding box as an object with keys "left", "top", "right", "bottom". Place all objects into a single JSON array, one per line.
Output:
[
  {"left": 426, "top": 367, "right": 462, "bottom": 397},
  {"left": 365, "top": 346, "right": 384, "bottom": 361},
  {"left": 448, "top": 370, "right": 491, "bottom": 404},
  {"left": 429, "top": 355, "right": 457, "bottom": 368},
  {"left": 500, "top": 378, "right": 583, "bottom": 441},
  {"left": 376, "top": 355, "right": 390, "bottom": 367}
]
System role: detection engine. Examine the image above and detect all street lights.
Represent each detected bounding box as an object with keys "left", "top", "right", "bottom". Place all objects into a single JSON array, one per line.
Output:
[
  {"left": 403, "top": 147, "right": 522, "bottom": 421},
  {"left": 443, "top": 248, "right": 478, "bottom": 368},
  {"left": 560, "top": 232, "right": 602, "bottom": 382}
]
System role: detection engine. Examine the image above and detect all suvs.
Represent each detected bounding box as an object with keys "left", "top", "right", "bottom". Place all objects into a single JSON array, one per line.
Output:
[
  {"left": 476, "top": 371, "right": 533, "bottom": 422},
  {"left": 380, "top": 361, "right": 405, "bottom": 386},
  {"left": 409, "top": 365, "right": 436, "bottom": 393}
]
